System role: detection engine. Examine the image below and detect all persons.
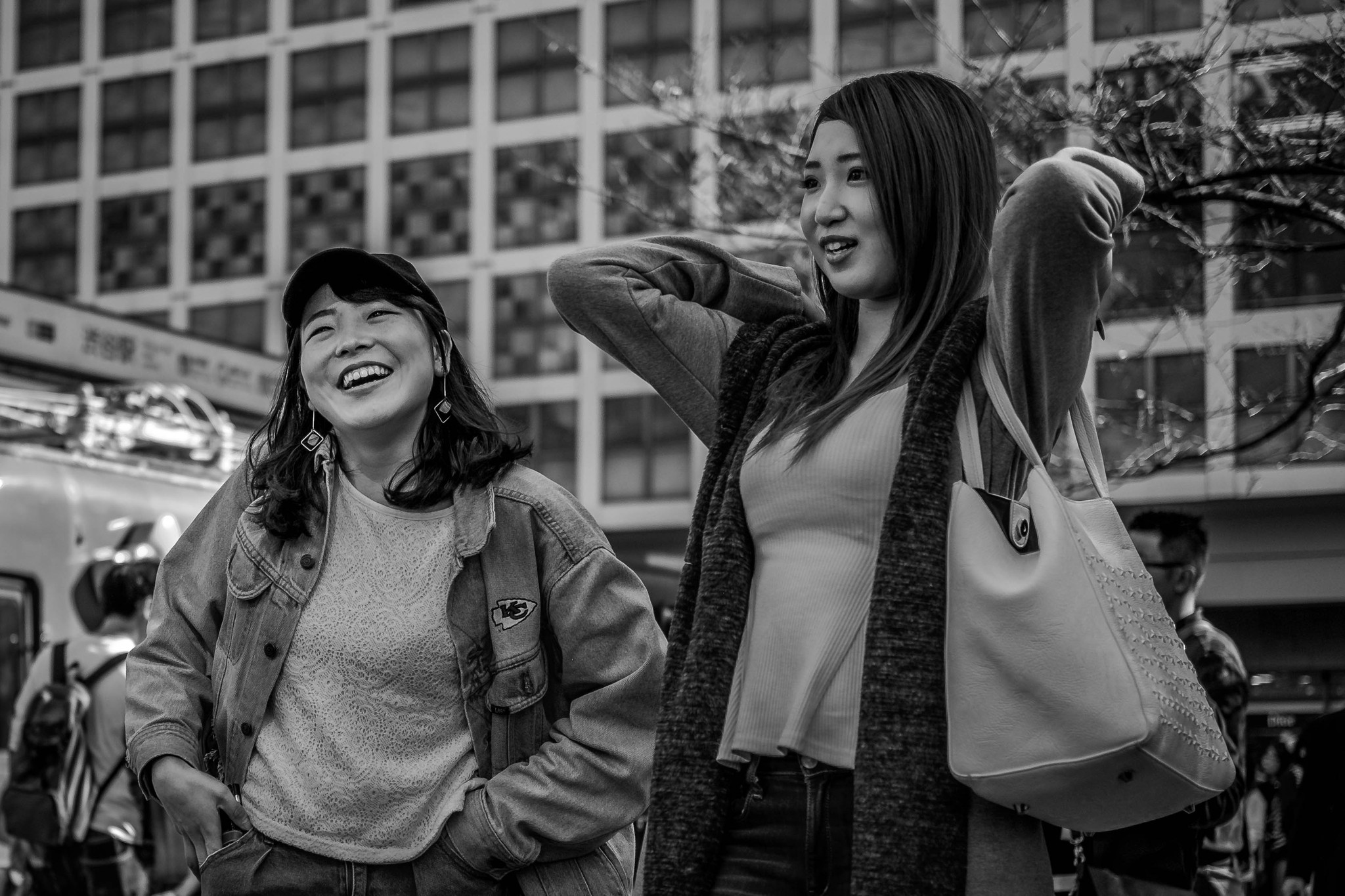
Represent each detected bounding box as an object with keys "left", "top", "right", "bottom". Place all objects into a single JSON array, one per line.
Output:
[
  {"left": 547, "top": 75, "right": 1145, "bottom": 896},
  {"left": 1074, "top": 510, "right": 1250, "bottom": 896},
  {"left": 125, "top": 247, "right": 667, "bottom": 895},
  {"left": 1, "top": 559, "right": 197, "bottom": 895}
]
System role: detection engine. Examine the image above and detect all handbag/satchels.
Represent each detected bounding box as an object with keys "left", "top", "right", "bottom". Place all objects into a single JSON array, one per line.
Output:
[{"left": 943, "top": 347, "right": 1236, "bottom": 834}]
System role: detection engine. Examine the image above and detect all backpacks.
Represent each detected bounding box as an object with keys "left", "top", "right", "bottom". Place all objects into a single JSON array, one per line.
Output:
[{"left": 0, "top": 641, "right": 138, "bottom": 846}]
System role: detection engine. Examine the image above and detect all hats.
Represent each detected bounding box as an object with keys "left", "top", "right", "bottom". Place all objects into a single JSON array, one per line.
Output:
[{"left": 282, "top": 247, "right": 448, "bottom": 351}]
